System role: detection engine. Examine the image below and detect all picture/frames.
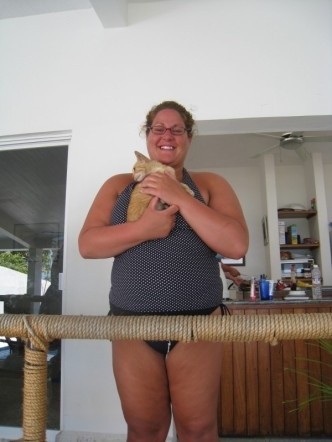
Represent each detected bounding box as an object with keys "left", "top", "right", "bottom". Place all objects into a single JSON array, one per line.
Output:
[
  {"left": 221, "top": 256, "right": 245, "bottom": 267},
  {"left": 261, "top": 216, "right": 269, "bottom": 246}
]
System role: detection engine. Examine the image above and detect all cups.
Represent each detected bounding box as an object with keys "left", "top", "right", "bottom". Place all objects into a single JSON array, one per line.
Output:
[{"left": 259, "top": 281, "right": 269, "bottom": 300}]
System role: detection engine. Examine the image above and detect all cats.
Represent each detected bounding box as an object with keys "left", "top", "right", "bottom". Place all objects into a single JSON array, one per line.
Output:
[{"left": 126, "top": 150, "right": 195, "bottom": 223}]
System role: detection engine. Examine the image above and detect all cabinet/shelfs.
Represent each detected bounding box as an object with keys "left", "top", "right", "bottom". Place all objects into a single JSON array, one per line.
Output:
[{"left": 277, "top": 209, "right": 320, "bottom": 287}]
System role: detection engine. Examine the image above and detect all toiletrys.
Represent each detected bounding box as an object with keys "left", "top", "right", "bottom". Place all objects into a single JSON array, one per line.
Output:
[
  {"left": 248, "top": 277, "right": 256, "bottom": 302},
  {"left": 259, "top": 274, "right": 275, "bottom": 300}
]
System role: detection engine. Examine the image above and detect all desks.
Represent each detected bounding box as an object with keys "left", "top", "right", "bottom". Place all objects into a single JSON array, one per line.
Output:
[
  {"left": 218, "top": 296, "right": 332, "bottom": 438},
  {"left": 248, "top": 133, "right": 332, "bottom": 162}
]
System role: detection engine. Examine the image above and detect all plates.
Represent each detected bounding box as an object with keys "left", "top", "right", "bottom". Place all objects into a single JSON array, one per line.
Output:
[
  {"left": 278, "top": 203, "right": 308, "bottom": 211},
  {"left": 284, "top": 290, "right": 309, "bottom": 300}
]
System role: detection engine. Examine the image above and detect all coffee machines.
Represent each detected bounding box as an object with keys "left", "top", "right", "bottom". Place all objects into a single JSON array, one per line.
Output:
[{"left": 311, "top": 265, "right": 323, "bottom": 300}]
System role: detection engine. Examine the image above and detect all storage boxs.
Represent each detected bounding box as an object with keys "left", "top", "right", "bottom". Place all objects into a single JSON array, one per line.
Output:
[{"left": 286, "top": 225, "right": 297, "bottom": 244}]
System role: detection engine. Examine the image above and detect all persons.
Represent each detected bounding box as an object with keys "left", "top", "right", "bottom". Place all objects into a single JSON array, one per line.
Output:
[
  {"left": 78, "top": 101, "right": 248, "bottom": 442},
  {"left": 216, "top": 253, "right": 242, "bottom": 301}
]
